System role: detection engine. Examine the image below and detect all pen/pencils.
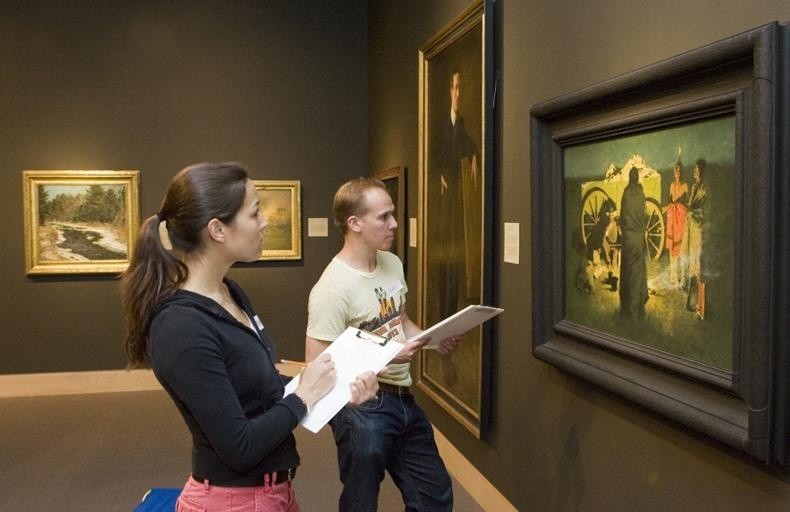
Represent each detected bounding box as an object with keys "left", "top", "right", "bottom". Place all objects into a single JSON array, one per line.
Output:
[{"left": 280, "top": 359, "right": 309, "bottom": 366}]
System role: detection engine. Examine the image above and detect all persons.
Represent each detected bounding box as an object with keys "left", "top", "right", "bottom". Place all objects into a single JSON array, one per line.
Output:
[
  {"left": 429, "top": 64, "right": 484, "bottom": 393},
  {"left": 113, "top": 160, "right": 392, "bottom": 511},
  {"left": 620, "top": 166, "right": 648, "bottom": 319},
  {"left": 304, "top": 175, "right": 464, "bottom": 511},
  {"left": 685, "top": 157, "right": 726, "bottom": 323},
  {"left": 662, "top": 159, "right": 690, "bottom": 282}
]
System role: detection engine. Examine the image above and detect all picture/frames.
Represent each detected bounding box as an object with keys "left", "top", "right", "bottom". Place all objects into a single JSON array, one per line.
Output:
[
  {"left": 414, "top": 2, "right": 496, "bottom": 445},
  {"left": 249, "top": 181, "right": 303, "bottom": 262},
  {"left": 529, "top": 21, "right": 777, "bottom": 469},
  {"left": 22, "top": 167, "right": 141, "bottom": 278},
  {"left": 371, "top": 166, "right": 408, "bottom": 265}
]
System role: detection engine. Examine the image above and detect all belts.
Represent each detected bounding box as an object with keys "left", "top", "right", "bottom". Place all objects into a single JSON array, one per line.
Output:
[
  {"left": 191, "top": 464, "right": 297, "bottom": 488},
  {"left": 378, "top": 381, "right": 411, "bottom": 397}
]
